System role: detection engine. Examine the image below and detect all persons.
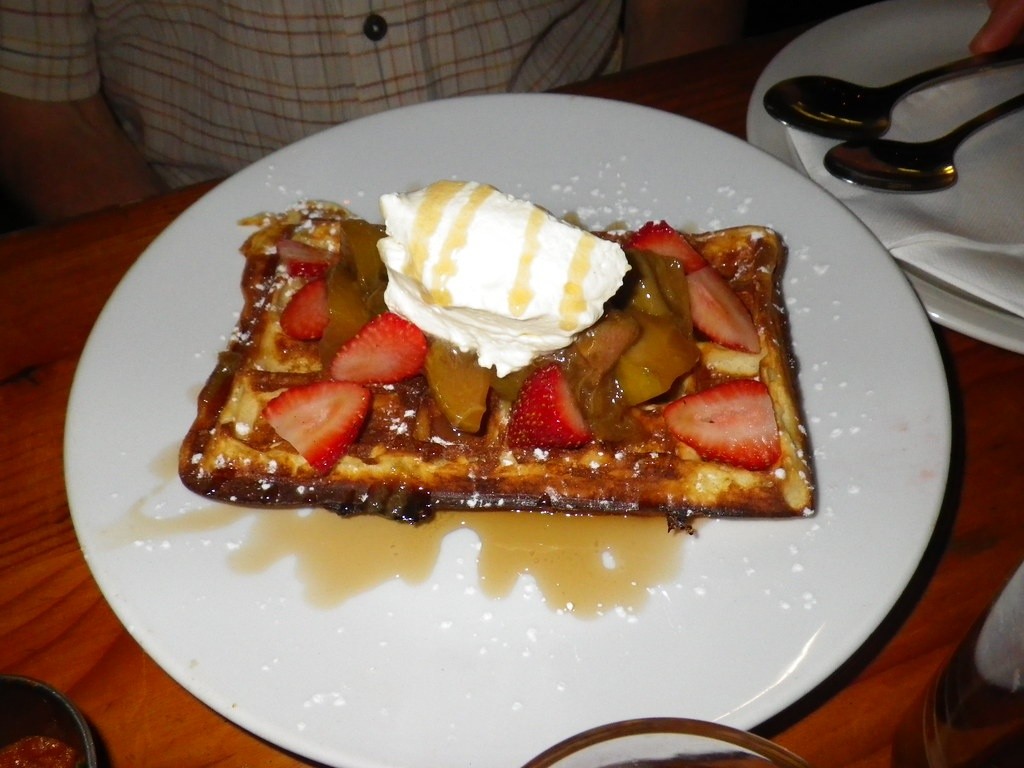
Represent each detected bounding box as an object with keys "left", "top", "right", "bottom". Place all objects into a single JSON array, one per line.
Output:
[
  {"left": 0, "top": 0, "right": 745, "bottom": 226},
  {"left": 969, "top": 0, "right": 1024, "bottom": 54}
]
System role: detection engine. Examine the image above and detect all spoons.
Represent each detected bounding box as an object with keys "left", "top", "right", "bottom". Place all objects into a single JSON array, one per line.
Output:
[
  {"left": 763, "top": 45, "right": 1023, "bottom": 141},
  {"left": 822, "top": 92, "right": 1022, "bottom": 194}
]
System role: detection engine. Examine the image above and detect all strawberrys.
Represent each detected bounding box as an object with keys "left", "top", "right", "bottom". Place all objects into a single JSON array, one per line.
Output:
[{"left": 266, "top": 218, "right": 784, "bottom": 478}]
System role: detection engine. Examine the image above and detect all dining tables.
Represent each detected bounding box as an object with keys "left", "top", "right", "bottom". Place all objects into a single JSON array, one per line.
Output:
[{"left": 0, "top": 29, "right": 1023, "bottom": 768}]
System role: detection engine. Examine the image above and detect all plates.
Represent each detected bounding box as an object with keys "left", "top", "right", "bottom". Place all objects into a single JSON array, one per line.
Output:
[
  {"left": 748, "top": 0, "right": 1024, "bottom": 358},
  {"left": 60, "top": 96, "right": 954, "bottom": 767}
]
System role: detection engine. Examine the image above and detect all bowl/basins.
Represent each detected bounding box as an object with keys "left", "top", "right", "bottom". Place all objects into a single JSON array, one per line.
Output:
[
  {"left": 525, "top": 717, "right": 811, "bottom": 766},
  {"left": 0, "top": 674, "right": 97, "bottom": 768}
]
